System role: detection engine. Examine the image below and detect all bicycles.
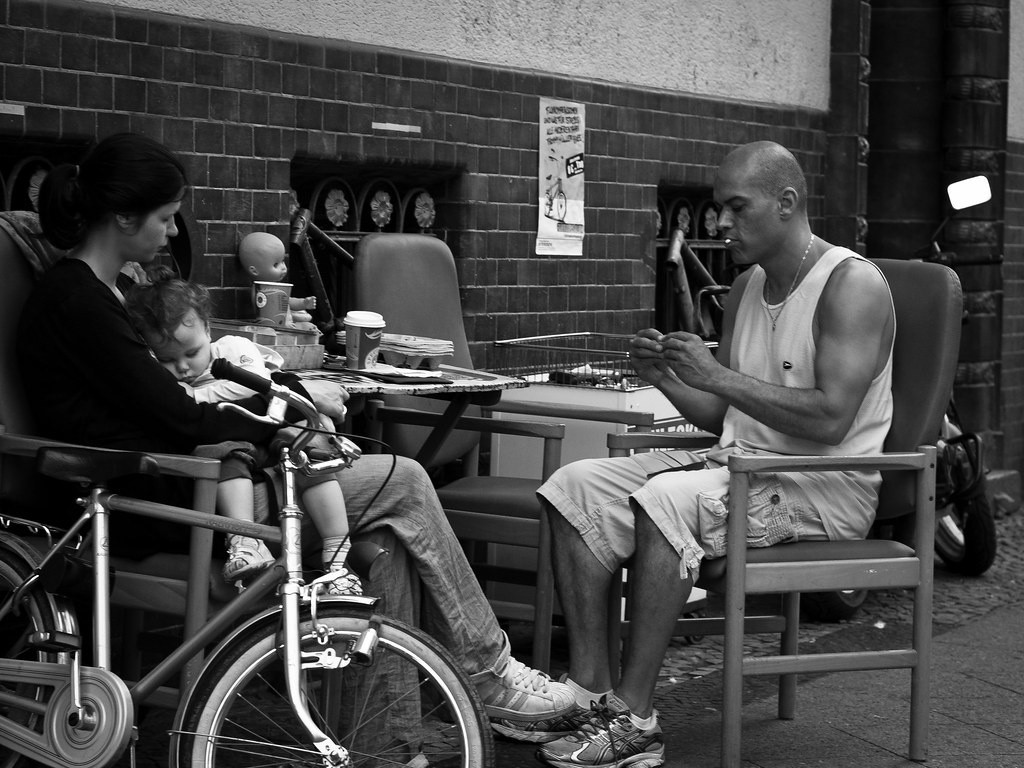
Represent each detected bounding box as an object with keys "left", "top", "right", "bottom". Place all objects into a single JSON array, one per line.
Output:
[{"left": 0, "top": 358, "right": 496, "bottom": 768}]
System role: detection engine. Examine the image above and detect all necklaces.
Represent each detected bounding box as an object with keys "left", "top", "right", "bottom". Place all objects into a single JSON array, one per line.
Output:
[{"left": 766, "top": 233, "right": 815, "bottom": 331}]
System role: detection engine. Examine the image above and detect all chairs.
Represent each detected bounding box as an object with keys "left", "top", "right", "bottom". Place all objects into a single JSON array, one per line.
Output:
[
  {"left": 356, "top": 234, "right": 654, "bottom": 686},
  {"left": 607, "top": 255, "right": 963, "bottom": 768},
  {"left": 1, "top": 209, "right": 343, "bottom": 758}
]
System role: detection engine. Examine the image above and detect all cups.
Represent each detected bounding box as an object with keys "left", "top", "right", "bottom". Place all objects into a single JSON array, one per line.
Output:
[
  {"left": 254, "top": 280, "right": 293, "bottom": 327},
  {"left": 343, "top": 310, "right": 385, "bottom": 372}
]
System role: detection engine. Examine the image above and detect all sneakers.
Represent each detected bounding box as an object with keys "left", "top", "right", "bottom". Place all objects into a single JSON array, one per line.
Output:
[
  {"left": 324, "top": 562, "right": 363, "bottom": 595},
  {"left": 490, "top": 671, "right": 614, "bottom": 742},
  {"left": 536, "top": 693, "right": 666, "bottom": 768},
  {"left": 469, "top": 628, "right": 577, "bottom": 723},
  {"left": 219, "top": 533, "right": 275, "bottom": 584}
]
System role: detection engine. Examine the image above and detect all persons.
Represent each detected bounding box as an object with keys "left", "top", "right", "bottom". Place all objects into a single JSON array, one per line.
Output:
[
  {"left": 10, "top": 132, "right": 576, "bottom": 768},
  {"left": 488, "top": 141, "right": 897, "bottom": 768},
  {"left": 237, "top": 231, "right": 321, "bottom": 335},
  {"left": 125, "top": 264, "right": 361, "bottom": 598}
]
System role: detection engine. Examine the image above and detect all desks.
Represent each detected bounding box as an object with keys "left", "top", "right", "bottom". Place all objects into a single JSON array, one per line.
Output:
[{"left": 277, "top": 365, "right": 534, "bottom": 496}]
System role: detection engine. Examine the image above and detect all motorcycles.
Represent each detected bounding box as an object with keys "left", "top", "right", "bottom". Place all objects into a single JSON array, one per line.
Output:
[{"left": 700, "top": 167, "right": 1000, "bottom": 621}]
